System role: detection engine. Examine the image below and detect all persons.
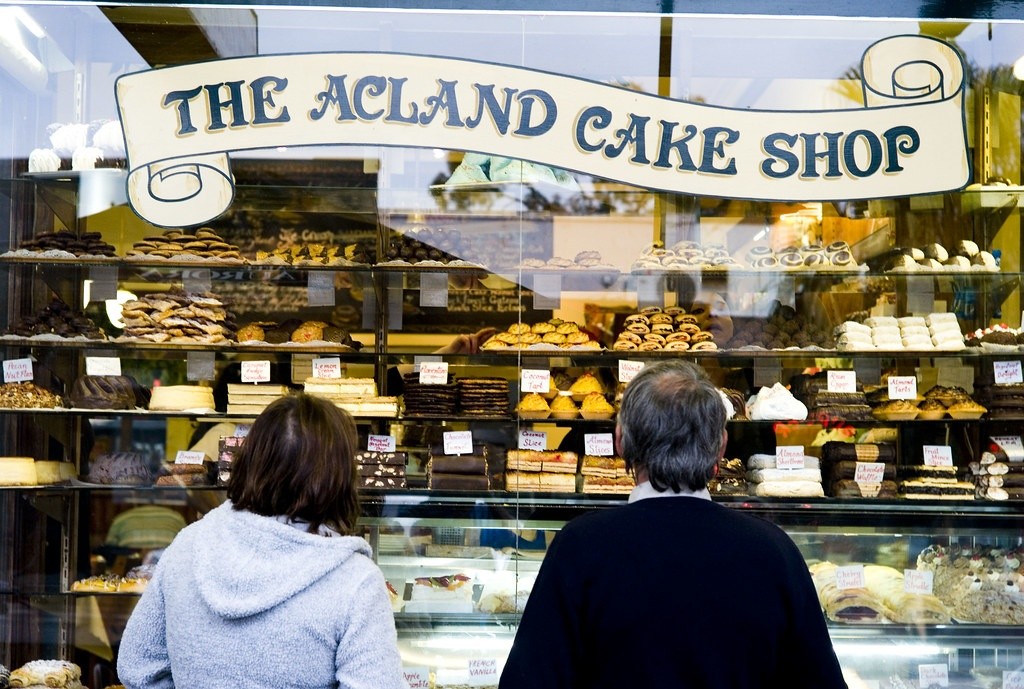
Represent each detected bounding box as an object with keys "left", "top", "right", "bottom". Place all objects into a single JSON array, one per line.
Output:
[
  {"left": 685, "top": 289, "right": 735, "bottom": 350},
  {"left": 497, "top": 361, "right": 850, "bottom": 689},
  {"left": 385, "top": 327, "right": 497, "bottom": 398},
  {"left": 117, "top": 391, "right": 405, "bottom": 689}
]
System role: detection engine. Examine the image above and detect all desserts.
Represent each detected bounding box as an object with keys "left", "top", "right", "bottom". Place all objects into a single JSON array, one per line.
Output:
[{"left": 0, "top": 225, "right": 1024, "bottom": 625}]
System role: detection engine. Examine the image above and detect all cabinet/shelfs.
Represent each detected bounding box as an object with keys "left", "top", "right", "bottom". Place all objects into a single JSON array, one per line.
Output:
[
  {"left": 377, "top": 31, "right": 689, "bottom": 689},
  {"left": 0, "top": 24, "right": 378, "bottom": 688},
  {"left": 687, "top": 35, "right": 1023, "bottom": 688}
]
